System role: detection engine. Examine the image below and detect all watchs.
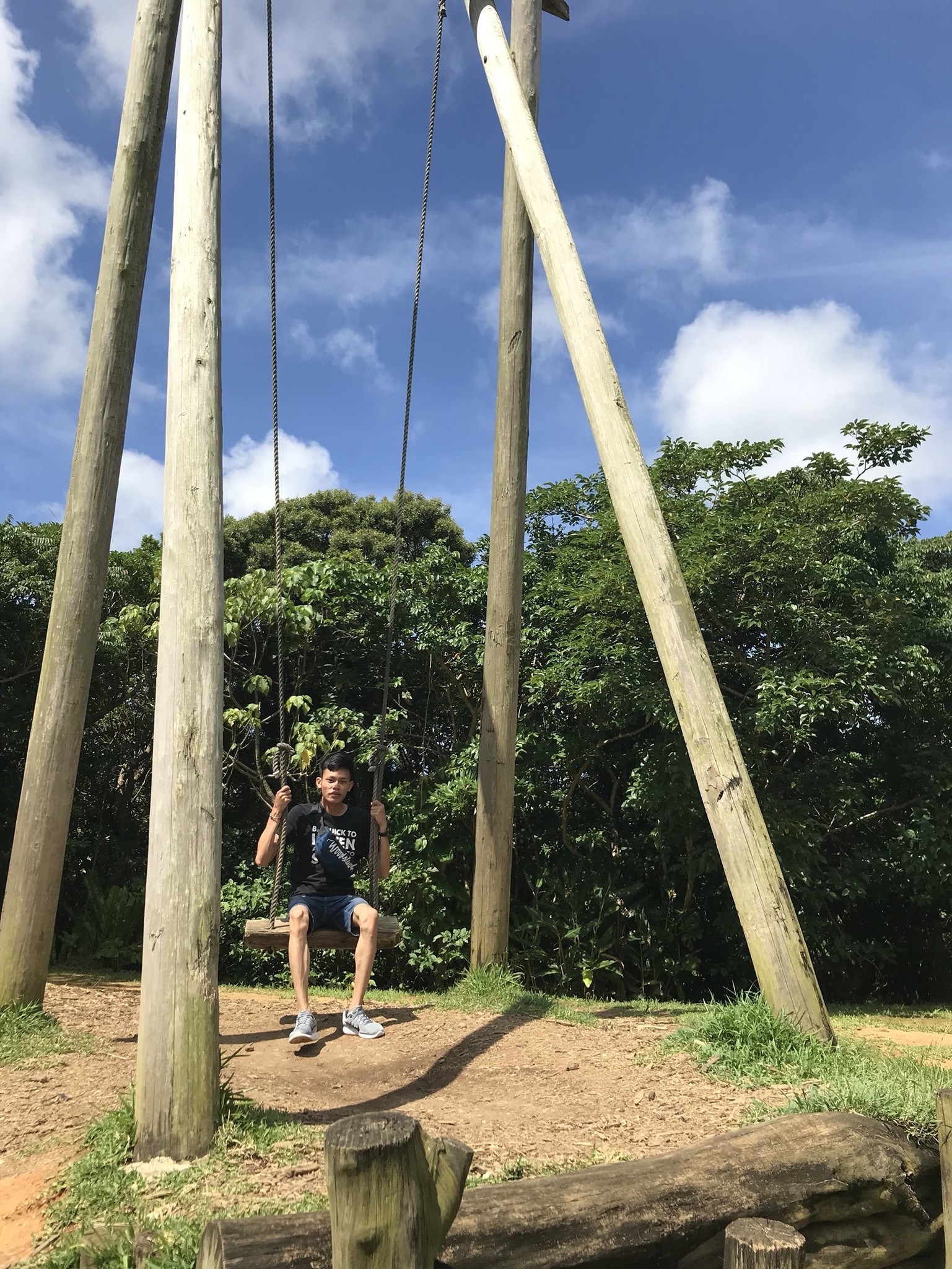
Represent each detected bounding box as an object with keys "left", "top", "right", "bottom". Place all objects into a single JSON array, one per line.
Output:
[{"left": 378, "top": 827, "right": 389, "bottom": 837}]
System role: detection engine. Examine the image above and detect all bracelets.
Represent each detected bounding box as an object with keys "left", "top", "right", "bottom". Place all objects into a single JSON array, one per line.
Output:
[{"left": 269, "top": 813, "right": 281, "bottom": 819}]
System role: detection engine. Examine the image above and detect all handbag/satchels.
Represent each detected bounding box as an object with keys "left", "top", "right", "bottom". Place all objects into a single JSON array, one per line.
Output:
[{"left": 314, "top": 828, "right": 359, "bottom": 880}]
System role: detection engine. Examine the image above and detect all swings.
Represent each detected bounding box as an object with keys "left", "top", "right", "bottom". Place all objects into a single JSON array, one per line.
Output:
[{"left": 240, "top": 0, "right": 452, "bottom": 950}]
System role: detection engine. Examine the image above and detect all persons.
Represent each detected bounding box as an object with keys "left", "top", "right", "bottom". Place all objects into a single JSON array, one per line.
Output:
[{"left": 255, "top": 752, "right": 390, "bottom": 1044}]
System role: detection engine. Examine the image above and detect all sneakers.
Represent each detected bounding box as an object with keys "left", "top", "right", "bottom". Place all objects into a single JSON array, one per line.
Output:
[
  {"left": 289, "top": 1009, "right": 318, "bottom": 1043},
  {"left": 342, "top": 1005, "right": 384, "bottom": 1039}
]
